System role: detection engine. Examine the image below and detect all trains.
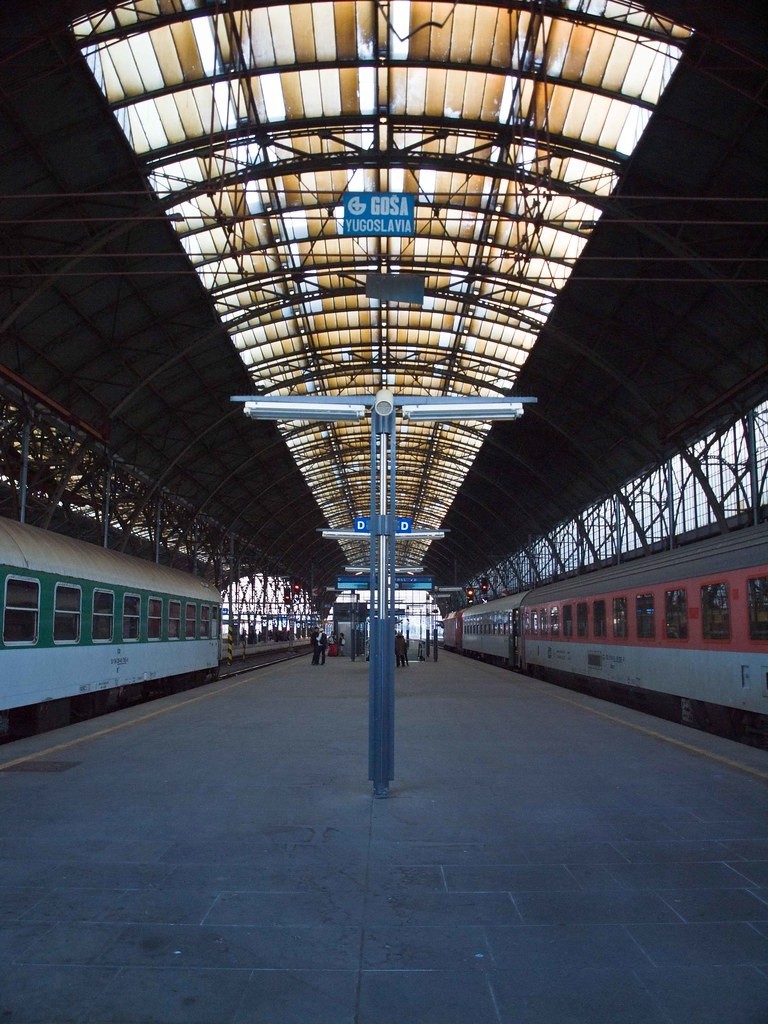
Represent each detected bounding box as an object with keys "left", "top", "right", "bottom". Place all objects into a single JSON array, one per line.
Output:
[
  {"left": 441, "top": 517, "right": 768, "bottom": 748},
  {"left": 0, "top": 516, "right": 223, "bottom": 736}
]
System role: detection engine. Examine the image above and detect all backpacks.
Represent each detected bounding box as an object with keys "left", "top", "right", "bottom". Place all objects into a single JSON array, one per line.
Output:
[
  {"left": 318, "top": 632, "right": 328, "bottom": 644},
  {"left": 340, "top": 637, "right": 346, "bottom": 646}
]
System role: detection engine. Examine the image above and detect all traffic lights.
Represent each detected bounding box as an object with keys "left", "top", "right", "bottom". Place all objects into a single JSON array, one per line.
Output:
[
  {"left": 284, "top": 588, "right": 291, "bottom": 604},
  {"left": 481, "top": 578, "right": 489, "bottom": 594},
  {"left": 466, "top": 587, "right": 474, "bottom": 605},
  {"left": 292, "top": 578, "right": 301, "bottom": 595}
]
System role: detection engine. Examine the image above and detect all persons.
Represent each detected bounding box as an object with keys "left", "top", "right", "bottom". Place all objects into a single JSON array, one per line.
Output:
[
  {"left": 310, "top": 627, "right": 327, "bottom": 666},
  {"left": 269, "top": 630, "right": 290, "bottom": 642},
  {"left": 328, "top": 631, "right": 345, "bottom": 657},
  {"left": 242, "top": 630, "right": 246, "bottom": 648},
  {"left": 395, "top": 631, "right": 409, "bottom": 667}
]
